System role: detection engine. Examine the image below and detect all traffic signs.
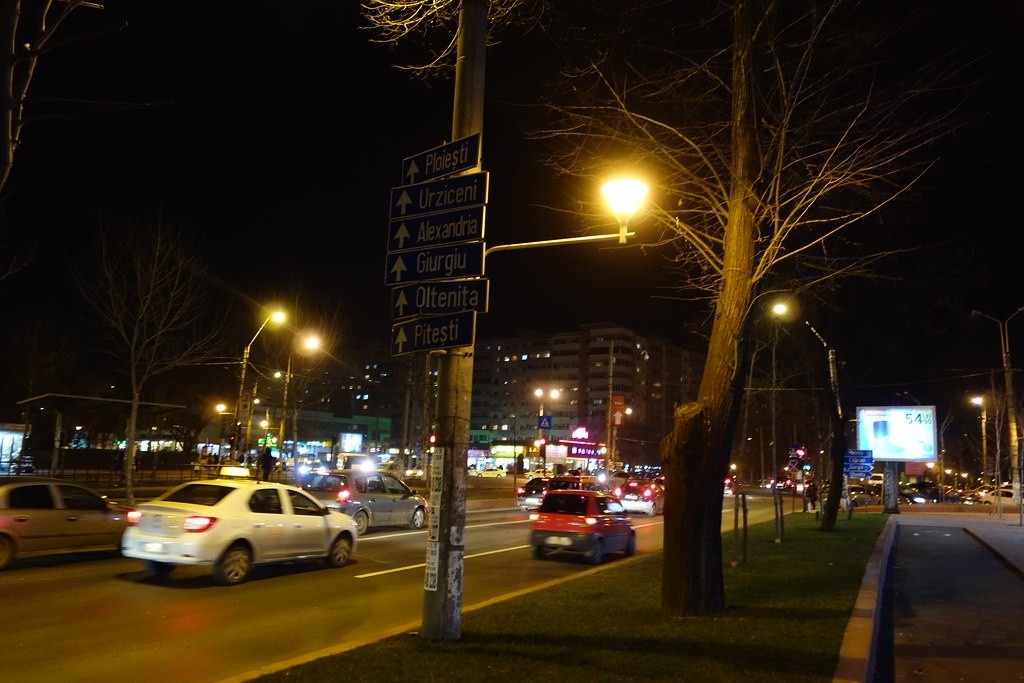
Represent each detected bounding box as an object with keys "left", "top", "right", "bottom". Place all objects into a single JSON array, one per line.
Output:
[
  {"left": 843, "top": 462, "right": 875, "bottom": 473},
  {"left": 389, "top": 309, "right": 478, "bottom": 358},
  {"left": 844, "top": 456, "right": 875, "bottom": 464},
  {"left": 388, "top": 171, "right": 490, "bottom": 219},
  {"left": 386, "top": 205, "right": 486, "bottom": 254},
  {"left": 383, "top": 241, "right": 487, "bottom": 287},
  {"left": 390, "top": 278, "right": 490, "bottom": 322},
  {"left": 844, "top": 448, "right": 873, "bottom": 458},
  {"left": 401, "top": 131, "right": 481, "bottom": 186},
  {"left": 842, "top": 470, "right": 872, "bottom": 479}
]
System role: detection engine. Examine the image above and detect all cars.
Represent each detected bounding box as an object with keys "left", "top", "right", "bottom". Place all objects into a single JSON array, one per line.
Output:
[
  {"left": 120, "top": 465, "right": 360, "bottom": 585},
  {"left": 474, "top": 466, "right": 507, "bottom": 478},
  {"left": 527, "top": 489, "right": 637, "bottom": 566},
  {"left": 764, "top": 473, "right": 1024, "bottom": 508},
  {"left": 292, "top": 468, "right": 429, "bottom": 537},
  {"left": 517, "top": 470, "right": 666, "bottom": 518},
  {"left": 0, "top": 480, "right": 137, "bottom": 572}
]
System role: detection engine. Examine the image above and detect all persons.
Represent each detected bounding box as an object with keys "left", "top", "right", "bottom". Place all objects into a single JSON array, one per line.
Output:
[
  {"left": 207, "top": 453, "right": 219, "bottom": 469},
  {"left": 239, "top": 454, "right": 244, "bottom": 463},
  {"left": 572, "top": 468, "right": 582, "bottom": 476},
  {"left": 344, "top": 458, "right": 351, "bottom": 469},
  {"left": 261, "top": 447, "right": 277, "bottom": 481},
  {"left": 247, "top": 456, "right": 252, "bottom": 464},
  {"left": 806, "top": 481, "right": 818, "bottom": 509}
]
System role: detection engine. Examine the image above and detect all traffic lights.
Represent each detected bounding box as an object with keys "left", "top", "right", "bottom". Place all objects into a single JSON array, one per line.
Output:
[{"left": 795, "top": 447, "right": 813, "bottom": 471}]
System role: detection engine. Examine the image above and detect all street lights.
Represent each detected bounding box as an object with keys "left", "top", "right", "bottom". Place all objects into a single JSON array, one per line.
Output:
[
  {"left": 415, "top": 163, "right": 655, "bottom": 644},
  {"left": 276, "top": 330, "right": 322, "bottom": 472},
  {"left": 968, "top": 394, "right": 988, "bottom": 484},
  {"left": 228, "top": 307, "right": 289, "bottom": 479},
  {"left": 533, "top": 385, "right": 562, "bottom": 469}
]
